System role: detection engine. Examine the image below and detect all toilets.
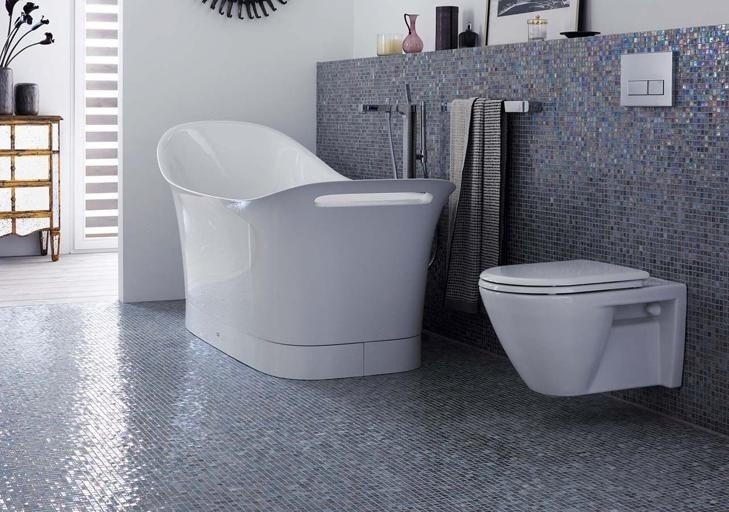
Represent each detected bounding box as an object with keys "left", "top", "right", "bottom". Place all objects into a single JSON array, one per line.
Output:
[{"left": 478, "top": 260, "right": 687, "bottom": 396}]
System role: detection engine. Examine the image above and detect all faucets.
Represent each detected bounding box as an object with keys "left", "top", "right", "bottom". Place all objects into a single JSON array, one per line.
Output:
[{"left": 359, "top": 104, "right": 397, "bottom": 115}]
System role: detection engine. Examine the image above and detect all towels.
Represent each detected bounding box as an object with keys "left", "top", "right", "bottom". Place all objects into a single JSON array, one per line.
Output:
[{"left": 437, "top": 96, "right": 512, "bottom": 320}]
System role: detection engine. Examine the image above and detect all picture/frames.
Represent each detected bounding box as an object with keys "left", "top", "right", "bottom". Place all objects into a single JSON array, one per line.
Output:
[{"left": 485, "top": 0, "right": 579, "bottom": 44}]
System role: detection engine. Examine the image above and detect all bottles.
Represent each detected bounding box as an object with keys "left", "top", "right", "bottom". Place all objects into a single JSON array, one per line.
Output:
[{"left": 401, "top": 13, "right": 424, "bottom": 54}]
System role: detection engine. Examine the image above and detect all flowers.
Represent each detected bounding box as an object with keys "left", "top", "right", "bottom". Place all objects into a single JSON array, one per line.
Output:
[{"left": 0, "top": 0, "right": 56, "bottom": 68}]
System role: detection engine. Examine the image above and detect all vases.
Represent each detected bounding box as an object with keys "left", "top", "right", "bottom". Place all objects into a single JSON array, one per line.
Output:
[{"left": 1, "top": 67, "right": 41, "bottom": 115}]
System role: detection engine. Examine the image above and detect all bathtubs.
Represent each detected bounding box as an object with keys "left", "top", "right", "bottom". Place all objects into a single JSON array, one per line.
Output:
[{"left": 157, "top": 119, "right": 456, "bottom": 381}]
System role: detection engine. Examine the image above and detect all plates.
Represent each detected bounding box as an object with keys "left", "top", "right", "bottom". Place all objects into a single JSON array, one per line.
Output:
[{"left": 560, "top": 31, "right": 600, "bottom": 38}]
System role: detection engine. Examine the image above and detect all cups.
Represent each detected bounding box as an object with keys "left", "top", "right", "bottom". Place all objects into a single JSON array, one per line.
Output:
[
  {"left": 376, "top": 32, "right": 404, "bottom": 56},
  {"left": 435, "top": 5, "right": 459, "bottom": 51},
  {"left": 527, "top": 18, "right": 548, "bottom": 41}
]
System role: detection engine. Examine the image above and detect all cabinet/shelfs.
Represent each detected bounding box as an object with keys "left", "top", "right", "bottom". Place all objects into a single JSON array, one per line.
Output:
[{"left": 0, "top": 116, "right": 65, "bottom": 263}]
